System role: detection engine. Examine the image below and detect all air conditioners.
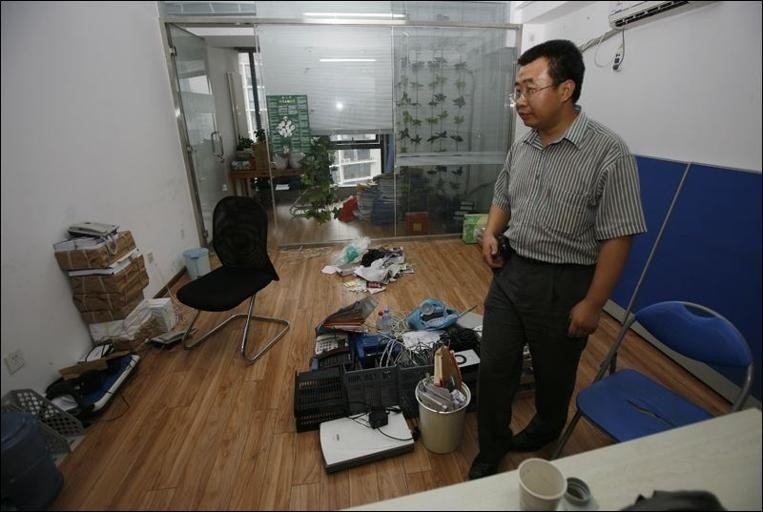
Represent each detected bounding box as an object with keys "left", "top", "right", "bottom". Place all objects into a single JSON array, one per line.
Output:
[{"left": 608, "top": 1, "right": 718, "bottom": 31}]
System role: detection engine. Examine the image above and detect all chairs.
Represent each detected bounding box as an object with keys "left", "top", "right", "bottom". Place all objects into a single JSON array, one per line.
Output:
[
  {"left": 176, "top": 195, "right": 290, "bottom": 365},
  {"left": 550, "top": 300, "right": 755, "bottom": 460}
]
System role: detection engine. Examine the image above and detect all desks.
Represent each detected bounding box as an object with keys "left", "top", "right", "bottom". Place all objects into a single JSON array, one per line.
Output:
[
  {"left": 338, "top": 407, "right": 762, "bottom": 512},
  {"left": 228, "top": 165, "right": 304, "bottom": 197}
]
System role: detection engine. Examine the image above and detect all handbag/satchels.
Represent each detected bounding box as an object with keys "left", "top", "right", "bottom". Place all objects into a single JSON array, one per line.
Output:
[
  {"left": 406, "top": 298, "right": 460, "bottom": 330},
  {"left": 495, "top": 233, "right": 513, "bottom": 258}
]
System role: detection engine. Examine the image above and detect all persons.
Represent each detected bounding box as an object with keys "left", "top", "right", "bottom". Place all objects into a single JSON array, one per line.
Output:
[{"left": 468, "top": 39, "right": 647, "bottom": 480}]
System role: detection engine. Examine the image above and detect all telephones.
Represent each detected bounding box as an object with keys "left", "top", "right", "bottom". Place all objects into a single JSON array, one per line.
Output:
[{"left": 67, "top": 221, "right": 119, "bottom": 237}]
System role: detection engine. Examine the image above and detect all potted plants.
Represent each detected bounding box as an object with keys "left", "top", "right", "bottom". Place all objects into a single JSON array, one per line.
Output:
[
  {"left": 251, "top": 128, "right": 273, "bottom": 156},
  {"left": 236, "top": 133, "right": 254, "bottom": 160}
]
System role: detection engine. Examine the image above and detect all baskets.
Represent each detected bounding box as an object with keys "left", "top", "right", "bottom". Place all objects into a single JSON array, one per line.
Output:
[
  {"left": 295, "top": 332, "right": 434, "bottom": 433},
  {"left": 2, "top": 388, "right": 87, "bottom": 455}
]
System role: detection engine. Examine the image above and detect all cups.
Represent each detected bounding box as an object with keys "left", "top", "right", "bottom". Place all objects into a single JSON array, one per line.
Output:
[{"left": 517, "top": 457, "right": 568, "bottom": 512}]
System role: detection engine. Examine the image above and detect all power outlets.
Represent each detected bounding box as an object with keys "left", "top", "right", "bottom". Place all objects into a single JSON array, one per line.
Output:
[
  {"left": 147, "top": 252, "right": 153, "bottom": 264},
  {"left": 3, "top": 347, "right": 25, "bottom": 374}
]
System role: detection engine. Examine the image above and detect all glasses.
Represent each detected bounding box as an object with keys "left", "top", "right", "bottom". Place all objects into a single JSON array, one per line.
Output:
[{"left": 514, "top": 82, "right": 560, "bottom": 101}]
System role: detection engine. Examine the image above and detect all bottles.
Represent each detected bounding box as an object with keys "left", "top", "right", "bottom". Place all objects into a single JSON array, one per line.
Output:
[{"left": 376, "top": 308, "right": 392, "bottom": 332}]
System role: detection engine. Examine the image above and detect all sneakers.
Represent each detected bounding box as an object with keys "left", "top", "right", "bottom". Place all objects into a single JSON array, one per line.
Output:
[{"left": 469, "top": 422, "right": 568, "bottom": 480}]
